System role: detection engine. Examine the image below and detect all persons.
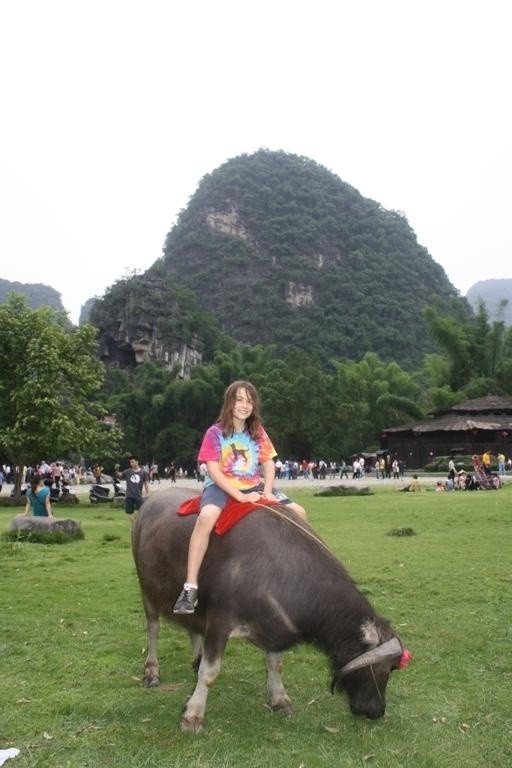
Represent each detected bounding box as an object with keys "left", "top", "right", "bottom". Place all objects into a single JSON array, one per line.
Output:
[
  {"left": 435, "top": 449, "right": 510, "bottom": 491},
  {"left": 141, "top": 462, "right": 205, "bottom": 486},
  {"left": 171, "top": 379, "right": 308, "bottom": 615},
  {"left": 16, "top": 475, "right": 54, "bottom": 520},
  {"left": 114, "top": 455, "right": 152, "bottom": 526},
  {"left": 274, "top": 453, "right": 401, "bottom": 480},
  {"left": 0, "top": 458, "right": 105, "bottom": 495},
  {"left": 392, "top": 474, "right": 425, "bottom": 493}
]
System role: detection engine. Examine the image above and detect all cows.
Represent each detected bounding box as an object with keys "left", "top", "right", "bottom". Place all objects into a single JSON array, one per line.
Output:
[{"left": 127, "top": 485, "right": 405, "bottom": 736}]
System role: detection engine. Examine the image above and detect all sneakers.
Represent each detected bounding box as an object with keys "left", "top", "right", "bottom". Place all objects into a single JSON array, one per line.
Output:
[{"left": 173, "top": 586, "right": 198, "bottom": 614}]
